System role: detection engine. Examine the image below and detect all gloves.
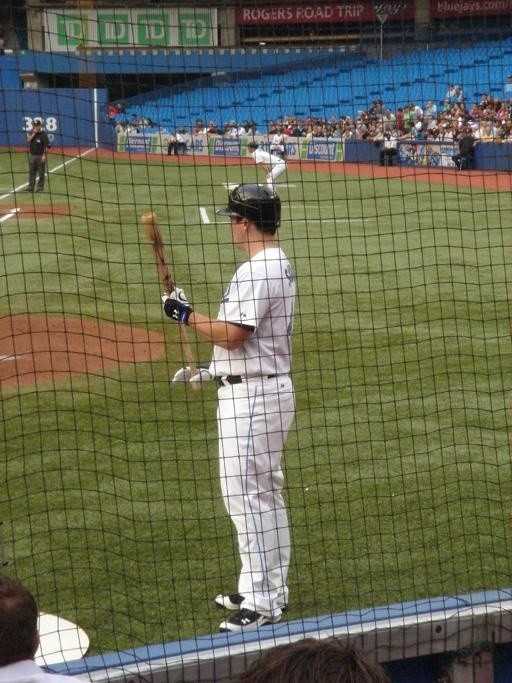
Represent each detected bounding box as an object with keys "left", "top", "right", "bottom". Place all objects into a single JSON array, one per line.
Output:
[
  {"left": 172, "top": 366, "right": 215, "bottom": 383},
  {"left": 161, "top": 286, "right": 193, "bottom": 326}
]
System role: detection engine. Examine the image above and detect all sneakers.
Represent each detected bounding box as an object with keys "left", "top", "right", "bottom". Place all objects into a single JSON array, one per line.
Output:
[
  {"left": 36, "top": 186, "right": 43, "bottom": 190},
  {"left": 219, "top": 608, "right": 282, "bottom": 632},
  {"left": 24, "top": 187, "right": 33, "bottom": 191},
  {"left": 215, "top": 593, "right": 288, "bottom": 611}
]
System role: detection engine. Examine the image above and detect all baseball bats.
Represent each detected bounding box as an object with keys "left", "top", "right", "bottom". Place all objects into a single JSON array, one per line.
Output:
[{"left": 142, "top": 213, "right": 200, "bottom": 390}]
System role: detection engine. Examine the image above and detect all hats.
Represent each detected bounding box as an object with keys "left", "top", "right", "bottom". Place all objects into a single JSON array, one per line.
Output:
[
  {"left": 32, "top": 120, "right": 41, "bottom": 126},
  {"left": 248, "top": 141, "right": 257, "bottom": 149}
]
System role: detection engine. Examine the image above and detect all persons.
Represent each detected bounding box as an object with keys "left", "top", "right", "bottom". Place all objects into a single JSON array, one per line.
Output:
[
  {"left": 23, "top": 120, "right": 51, "bottom": 192},
  {"left": 246, "top": 142, "right": 288, "bottom": 193},
  {"left": 268, "top": 111, "right": 369, "bottom": 140},
  {"left": 368, "top": 83, "right": 512, "bottom": 143},
  {"left": 0, "top": 576, "right": 89, "bottom": 683},
  {"left": 379, "top": 129, "right": 398, "bottom": 166},
  {"left": 162, "top": 184, "right": 298, "bottom": 633},
  {"left": 271, "top": 129, "right": 292, "bottom": 159},
  {"left": 115, "top": 113, "right": 153, "bottom": 136},
  {"left": 194, "top": 119, "right": 258, "bottom": 137},
  {"left": 452, "top": 128, "right": 481, "bottom": 170},
  {"left": 229, "top": 637, "right": 392, "bottom": 683},
  {"left": 167, "top": 127, "right": 191, "bottom": 156}
]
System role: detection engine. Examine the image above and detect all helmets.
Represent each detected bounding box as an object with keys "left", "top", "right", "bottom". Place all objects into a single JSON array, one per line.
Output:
[{"left": 216, "top": 182, "right": 281, "bottom": 228}]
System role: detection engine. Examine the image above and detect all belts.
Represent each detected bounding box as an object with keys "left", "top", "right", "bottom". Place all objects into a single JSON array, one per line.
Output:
[{"left": 215, "top": 371, "right": 275, "bottom": 385}]
